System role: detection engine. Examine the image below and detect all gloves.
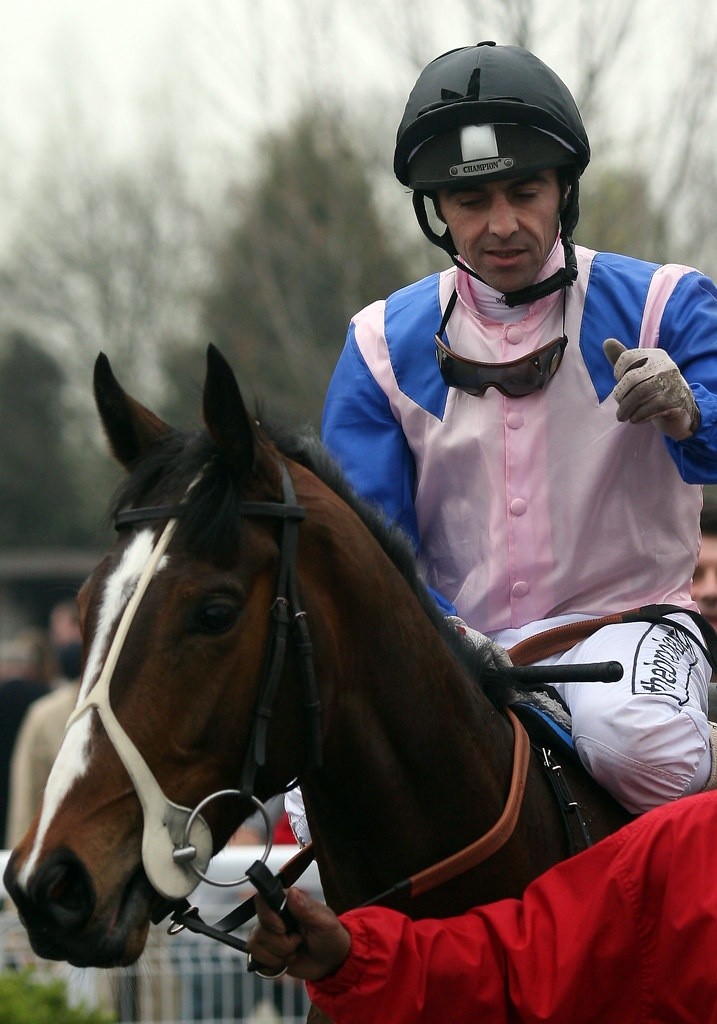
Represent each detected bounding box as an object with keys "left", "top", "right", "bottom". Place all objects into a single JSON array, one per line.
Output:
[
  {"left": 444, "top": 616, "right": 515, "bottom": 673},
  {"left": 602, "top": 338, "right": 700, "bottom": 442}
]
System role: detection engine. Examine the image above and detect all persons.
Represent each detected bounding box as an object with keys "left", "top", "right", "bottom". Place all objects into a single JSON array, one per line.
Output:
[
  {"left": 248, "top": 791, "right": 717, "bottom": 1024},
  {"left": 8, "top": 600, "right": 86, "bottom": 852},
  {"left": 284, "top": 41, "right": 717, "bottom": 848}
]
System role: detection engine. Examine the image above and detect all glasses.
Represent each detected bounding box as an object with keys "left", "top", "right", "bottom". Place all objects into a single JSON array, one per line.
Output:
[{"left": 433, "top": 284, "right": 568, "bottom": 399}]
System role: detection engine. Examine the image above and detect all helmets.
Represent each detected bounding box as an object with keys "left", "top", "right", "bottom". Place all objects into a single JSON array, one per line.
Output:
[{"left": 394, "top": 41, "right": 591, "bottom": 198}]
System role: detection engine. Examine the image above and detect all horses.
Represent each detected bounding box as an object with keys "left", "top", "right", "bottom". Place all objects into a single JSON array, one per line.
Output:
[{"left": 0, "top": 339, "right": 715, "bottom": 972}]
